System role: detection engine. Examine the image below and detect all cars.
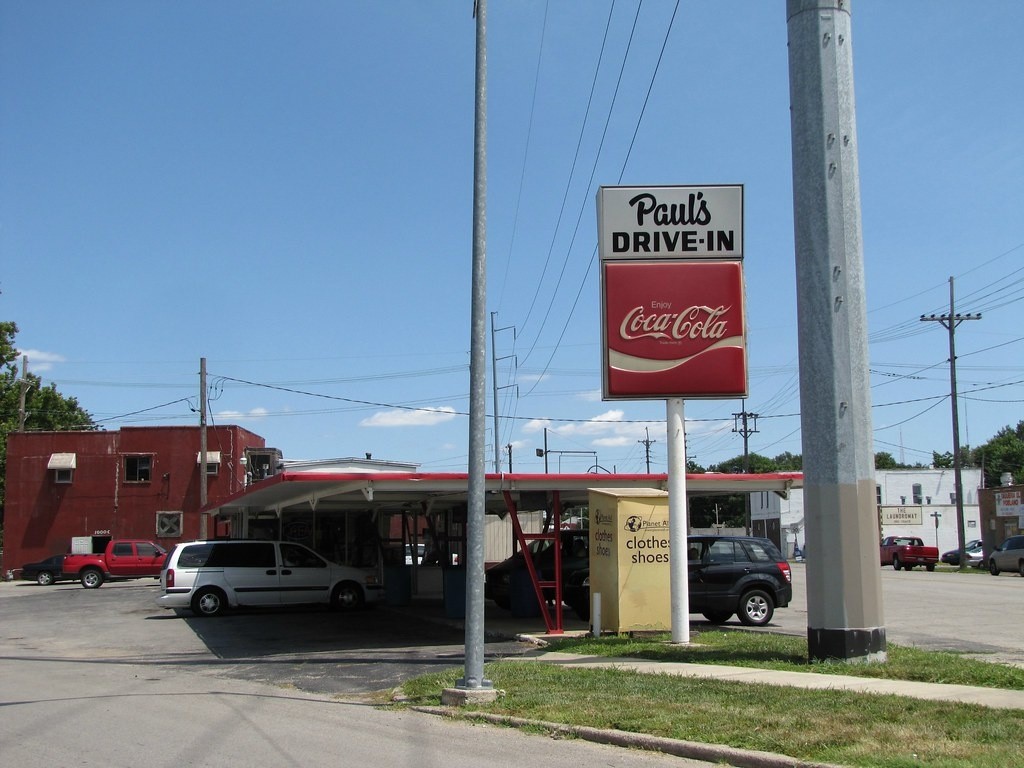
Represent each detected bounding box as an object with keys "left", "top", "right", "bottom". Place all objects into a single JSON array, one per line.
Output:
[
  {"left": 941, "top": 540, "right": 984, "bottom": 569},
  {"left": 19, "top": 554, "right": 63, "bottom": 585},
  {"left": 988, "top": 535, "right": 1024, "bottom": 576},
  {"left": 965, "top": 546, "right": 984, "bottom": 568}
]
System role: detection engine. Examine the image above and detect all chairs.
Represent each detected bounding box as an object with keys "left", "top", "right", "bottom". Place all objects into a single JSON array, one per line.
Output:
[
  {"left": 689, "top": 547, "right": 699, "bottom": 560},
  {"left": 572, "top": 539, "right": 586, "bottom": 557}
]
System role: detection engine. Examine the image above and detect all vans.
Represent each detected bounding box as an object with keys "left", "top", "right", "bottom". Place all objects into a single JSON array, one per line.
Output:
[{"left": 484, "top": 530, "right": 589, "bottom": 609}]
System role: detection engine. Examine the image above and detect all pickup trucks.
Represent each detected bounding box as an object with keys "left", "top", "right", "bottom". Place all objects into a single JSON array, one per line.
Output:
[
  {"left": 880, "top": 536, "right": 939, "bottom": 571},
  {"left": 63, "top": 540, "right": 169, "bottom": 588}
]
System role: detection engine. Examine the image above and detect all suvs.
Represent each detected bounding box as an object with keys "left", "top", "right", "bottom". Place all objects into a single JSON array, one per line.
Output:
[
  {"left": 154, "top": 535, "right": 387, "bottom": 617},
  {"left": 562, "top": 536, "right": 793, "bottom": 626}
]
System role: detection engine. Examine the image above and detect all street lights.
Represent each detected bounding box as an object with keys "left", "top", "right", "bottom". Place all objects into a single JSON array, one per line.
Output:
[{"left": 712, "top": 503, "right": 722, "bottom": 526}]
